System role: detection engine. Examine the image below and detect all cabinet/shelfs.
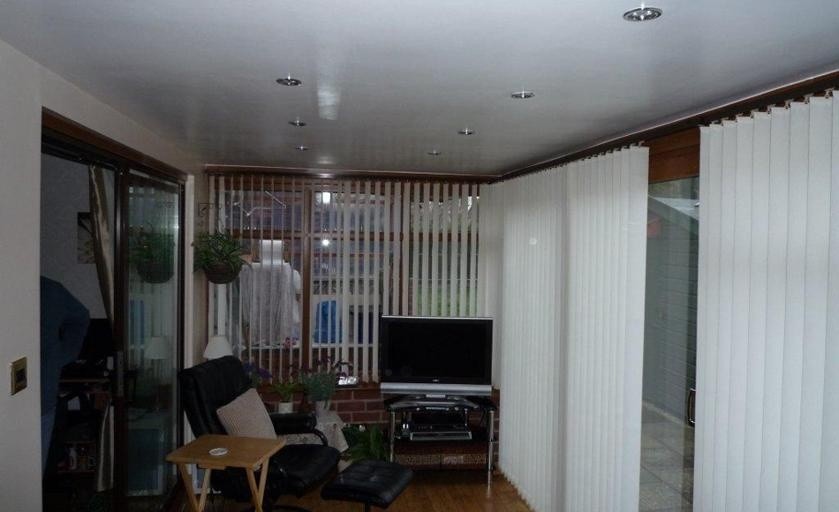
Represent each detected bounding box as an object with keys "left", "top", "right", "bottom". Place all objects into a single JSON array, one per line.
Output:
[{"left": 386, "top": 396, "right": 498, "bottom": 489}]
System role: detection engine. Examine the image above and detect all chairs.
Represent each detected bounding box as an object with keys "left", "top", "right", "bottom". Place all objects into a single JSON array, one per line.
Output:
[{"left": 177, "top": 355, "right": 341, "bottom": 511}]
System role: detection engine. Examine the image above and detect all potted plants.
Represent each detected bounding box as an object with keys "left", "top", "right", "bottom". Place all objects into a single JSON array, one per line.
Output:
[
  {"left": 260, "top": 364, "right": 302, "bottom": 414},
  {"left": 304, "top": 357, "right": 353, "bottom": 415},
  {"left": 129, "top": 230, "right": 176, "bottom": 283},
  {"left": 191, "top": 228, "right": 253, "bottom": 284}
]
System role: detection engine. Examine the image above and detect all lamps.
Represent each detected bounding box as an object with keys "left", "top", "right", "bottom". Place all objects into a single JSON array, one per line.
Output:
[
  {"left": 203, "top": 336, "right": 232, "bottom": 361},
  {"left": 144, "top": 335, "right": 172, "bottom": 379}
]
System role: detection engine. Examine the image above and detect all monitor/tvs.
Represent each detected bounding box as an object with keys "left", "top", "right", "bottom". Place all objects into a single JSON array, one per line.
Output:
[{"left": 377, "top": 314, "right": 495, "bottom": 396}]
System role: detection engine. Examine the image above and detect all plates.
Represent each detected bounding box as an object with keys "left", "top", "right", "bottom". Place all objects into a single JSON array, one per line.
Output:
[{"left": 209, "top": 448, "right": 228, "bottom": 455}]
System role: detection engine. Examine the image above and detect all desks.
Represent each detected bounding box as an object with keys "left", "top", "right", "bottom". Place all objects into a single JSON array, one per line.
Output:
[{"left": 166, "top": 436, "right": 288, "bottom": 511}]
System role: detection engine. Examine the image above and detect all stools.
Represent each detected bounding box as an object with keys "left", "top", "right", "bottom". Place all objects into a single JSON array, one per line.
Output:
[{"left": 321, "top": 458, "right": 414, "bottom": 512}]
